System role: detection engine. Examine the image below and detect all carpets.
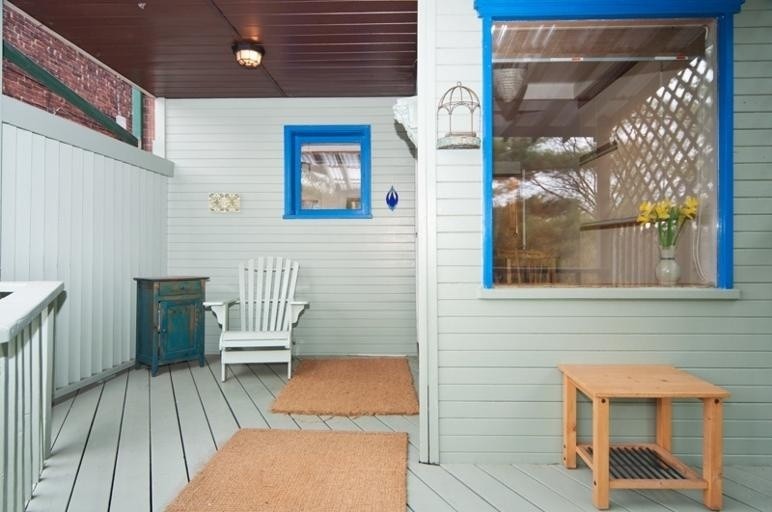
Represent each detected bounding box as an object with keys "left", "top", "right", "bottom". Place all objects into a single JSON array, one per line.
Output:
[
  {"left": 270, "top": 356, "right": 420, "bottom": 415},
  {"left": 163, "top": 427, "right": 408, "bottom": 511}
]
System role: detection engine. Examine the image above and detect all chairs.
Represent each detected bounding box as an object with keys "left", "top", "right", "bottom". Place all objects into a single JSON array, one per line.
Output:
[
  {"left": 201, "top": 255, "right": 312, "bottom": 382},
  {"left": 493, "top": 245, "right": 557, "bottom": 286}
]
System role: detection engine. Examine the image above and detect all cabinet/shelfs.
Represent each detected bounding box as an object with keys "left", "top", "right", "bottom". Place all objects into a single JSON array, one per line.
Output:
[{"left": 135, "top": 274, "right": 211, "bottom": 376}]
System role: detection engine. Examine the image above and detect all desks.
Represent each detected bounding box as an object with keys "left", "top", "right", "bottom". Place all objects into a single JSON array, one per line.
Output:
[{"left": 558, "top": 364, "right": 727, "bottom": 508}]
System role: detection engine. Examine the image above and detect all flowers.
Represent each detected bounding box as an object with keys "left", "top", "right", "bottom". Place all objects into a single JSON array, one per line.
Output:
[{"left": 632, "top": 197, "right": 702, "bottom": 248}]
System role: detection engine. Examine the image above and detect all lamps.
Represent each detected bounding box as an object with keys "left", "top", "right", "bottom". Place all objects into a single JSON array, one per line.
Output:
[
  {"left": 229, "top": 41, "right": 267, "bottom": 68},
  {"left": 490, "top": 60, "right": 531, "bottom": 104}
]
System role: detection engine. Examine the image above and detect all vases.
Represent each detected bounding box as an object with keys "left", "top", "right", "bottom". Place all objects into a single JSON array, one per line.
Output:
[{"left": 657, "top": 243, "right": 679, "bottom": 286}]
